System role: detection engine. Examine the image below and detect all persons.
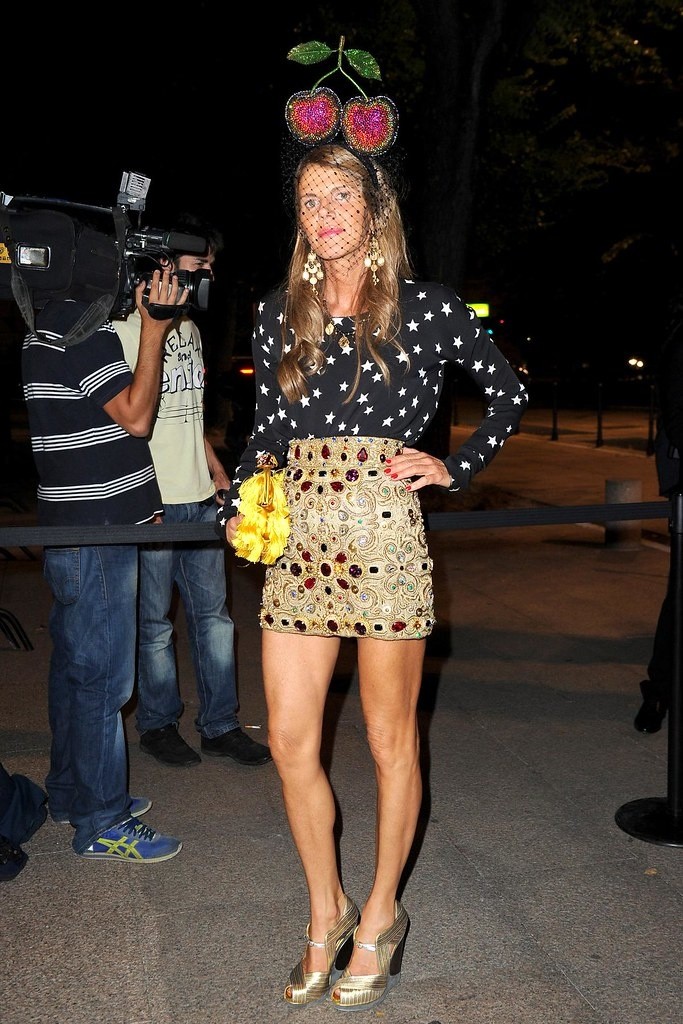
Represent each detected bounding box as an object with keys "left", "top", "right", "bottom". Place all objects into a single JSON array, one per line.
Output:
[
  {"left": 214, "top": 145, "right": 526, "bottom": 1009},
  {"left": 21, "top": 222, "right": 274, "bottom": 864}
]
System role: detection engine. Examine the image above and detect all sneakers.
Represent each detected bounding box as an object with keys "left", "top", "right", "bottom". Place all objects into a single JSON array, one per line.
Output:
[
  {"left": 200, "top": 726, "right": 273, "bottom": 766},
  {"left": 139, "top": 723, "right": 201, "bottom": 769},
  {"left": 79, "top": 816, "right": 183, "bottom": 863},
  {"left": 52, "top": 795, "right": 152, "bottom": 825}
]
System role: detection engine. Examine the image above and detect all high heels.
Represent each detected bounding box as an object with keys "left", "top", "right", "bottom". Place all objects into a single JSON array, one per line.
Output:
[{"left": 284, "top": 895, "right": 410, "bottom": 1012}]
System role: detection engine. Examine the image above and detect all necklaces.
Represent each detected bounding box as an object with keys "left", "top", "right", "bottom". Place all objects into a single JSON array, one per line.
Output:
[{"left": 323, "top": 288, "right": 377, "bottom": 349}]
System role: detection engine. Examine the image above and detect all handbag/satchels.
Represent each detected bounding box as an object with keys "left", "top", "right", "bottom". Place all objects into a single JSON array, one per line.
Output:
[{"left": 235, "top": 454, "right": 290, "bottom": 564}]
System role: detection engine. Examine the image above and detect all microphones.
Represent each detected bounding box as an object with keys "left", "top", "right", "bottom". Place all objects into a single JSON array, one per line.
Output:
[{"left": 133, "top": 231, "right": 206, "bottom": 252}]
